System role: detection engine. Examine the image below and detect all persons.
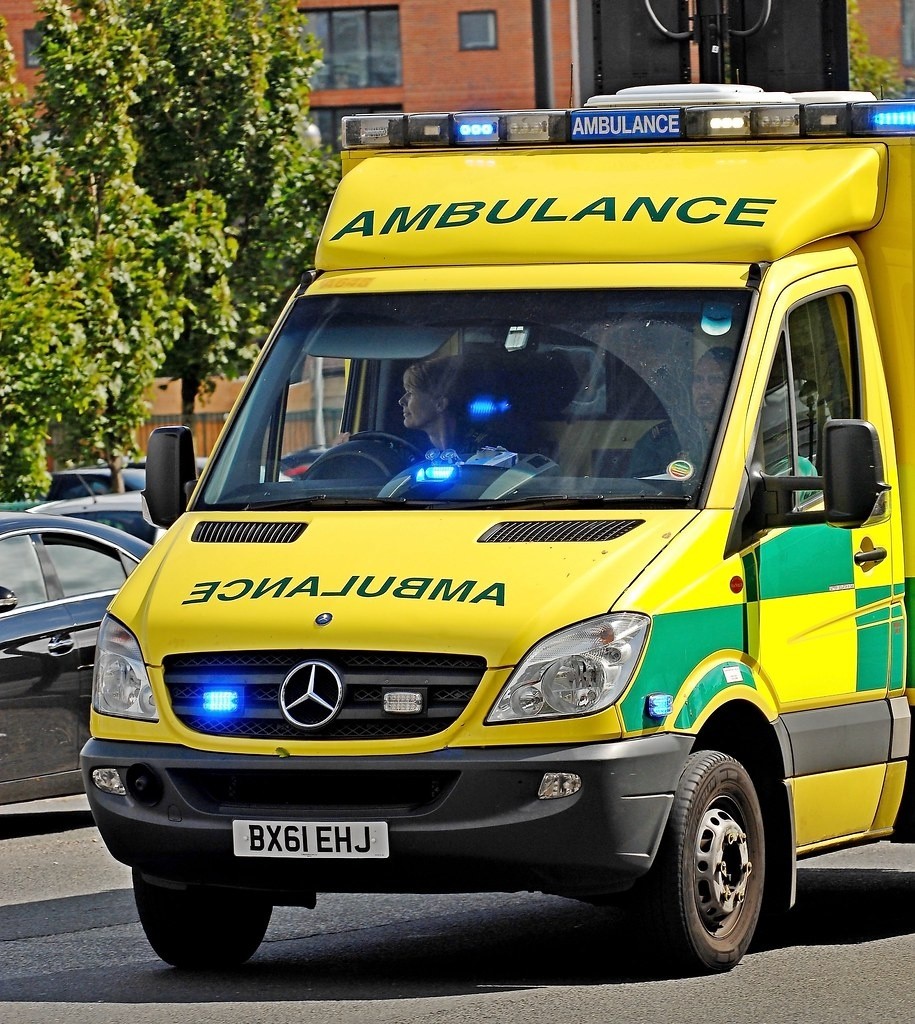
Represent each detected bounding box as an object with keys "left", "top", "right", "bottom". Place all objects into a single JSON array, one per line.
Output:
[
  {"left": 335, "top": 357, "right": 501, "bottom": 461},
  {"left": 621, "top": 337, "right": 733, "bottom": 477}
]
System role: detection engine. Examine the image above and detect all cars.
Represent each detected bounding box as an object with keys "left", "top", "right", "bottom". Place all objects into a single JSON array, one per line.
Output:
[
  {"left": 31, "top": 445, "right": 331, "bottom": 548},
  {"left": 1, "top": 511, "right": 157, "bottom": 815}
]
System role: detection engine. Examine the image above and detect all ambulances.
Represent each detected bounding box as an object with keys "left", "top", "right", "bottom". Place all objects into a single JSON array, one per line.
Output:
[{"left": 81, "top": 79, "right": 915, "bottom": 975}]
